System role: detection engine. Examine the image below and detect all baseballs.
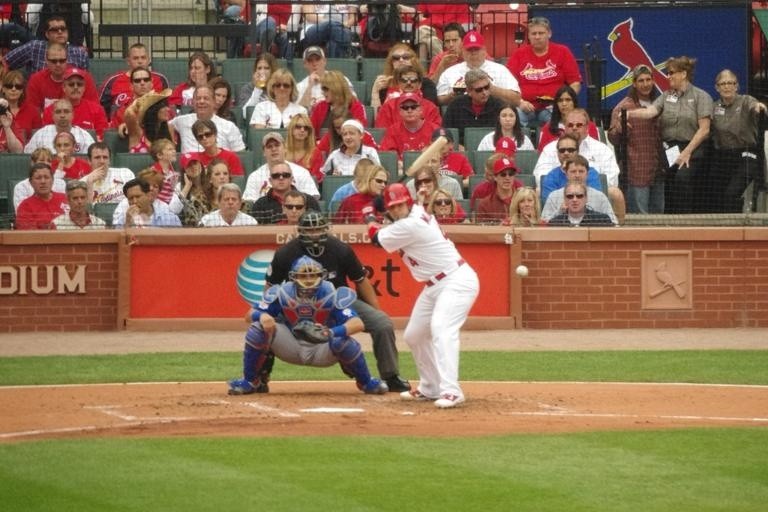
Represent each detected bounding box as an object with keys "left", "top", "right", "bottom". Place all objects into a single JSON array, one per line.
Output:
[{"left": 516, "top": 265, "right": 528, "bottom": 277}]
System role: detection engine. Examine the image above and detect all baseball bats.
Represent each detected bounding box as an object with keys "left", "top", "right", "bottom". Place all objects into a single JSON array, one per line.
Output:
[{"left": 374, "top": 136, "right": 448, "bottom": 206}]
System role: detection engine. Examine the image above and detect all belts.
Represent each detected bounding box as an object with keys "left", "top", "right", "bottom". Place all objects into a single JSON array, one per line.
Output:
[{"left": 426, "top": 258, "right": 465, "bottom": 286}]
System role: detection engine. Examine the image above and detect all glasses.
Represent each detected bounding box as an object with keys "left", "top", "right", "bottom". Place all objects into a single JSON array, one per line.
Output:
[
  {"left": 568, "top": 123, "right": 583, "bottom": 127},
  {"left": 453, "top": 77, "right": 466, "bottom": 92},
  {"left": 284, "top": 205, "right": 304, "bottom": 210},
  {"left": 566, "top": 195, "right": 583, "bottom": 199},
  {"left": 500, "top": 170, "right": 515, "bottom": 176},
  {"left": 371, "top": 178, "right": 387, "bottom": 185},
  {"left": 322, "top": 87, "right": 329, "bottom": 92},
  {"left": 272, "top": 173, "right": 291, "bottom": 179},
  {"left": 471, "top": 83, "right": 489, "bottom": 92},
  {"left": 434, "top": 199, "right": 451, "bottom": 205},
  {"left": 559, "top": 148, "right": 576, "bottom": 153},
  {"left": 132, "top": 78, "right": 150, "bottom": 83},
  {"left": 392, "top": 54, "right": 409, "bottom": 60},
  {"left": 48, "top": 25, "right": 66, "bottom": 34},
  {"left": 46, "top": 54, "right": 67, "bottom": 64},
  {"left": 399, "top": 77, "right": 418, "bottom": 83},
  {"left": 198, "top": 132, "right": 213, "bottom": 140},
  {"left": 417, "top": 176, "right": 432, "bottom": 185},
  {"left": 68, "top": 82, "right": 84, "bottom": 86},
  {"left": 536, "top": 96, "right": 554, "bottom": 103},
  {"left": 400, "top": 105, "right": 420, "bottom": 110},
  {"left": 666, "top": 71, "right": 676, "bottom": 75},
  {"left": 276, "top": 83, "right": 289, "bottom": 88},
  {"left": 295, "top": 126, "right": 308, "bottom": 131},
  {"left": 54, "top": 109, "right": 72, "bottom": 113},
  {"left": 717, "top": 80, "right": 735, "bottom": 88},
  {"left": 3, "top": 84, "right": 24, "bottom": 89}
]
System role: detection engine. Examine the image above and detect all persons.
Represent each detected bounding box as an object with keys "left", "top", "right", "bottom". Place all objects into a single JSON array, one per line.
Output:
[
  {"left": 436, "top": 128, "right": 475, "bottom": 200},
  {"left": 184, "top": 158, "right": 232, "bottom": 227},
  {"left": 198, "top": 183, "right": 258, "bottom": 227},
  {"left": 374, "top": 64, "right": 442, "bottom": 129},
  {"left": 146, "top": 139, "right": 181, "bottom": 204},
  {"left": 535, "top": 87, "right": 598, "bottom": 154},
  {"left": 442, "top": 69, "right": 508, "bottom": 154},
  {"left": 221, "top": 0, "right": 293, "bottom": 62},
  {"left": 48, "top": 179, "right": 109, "bottom": 231},
  {"left": 428, "top": 21, "right": 493, "bottom": 85},
  {"left": 13, "top": 147, "right": 66, "bottom": 217},
  {"left": 181, "top": 118, "right": 244, "bottom": 178},
  {"left": 25, "top": 44, "right": 100, "bottom": 111},
  {"left": 294, "top": 45, "right": 353, "bottom": 108},
  {"left": 546, "top": 180, "right": 616, "bottom": 228},
  {"left": 23, "top": 98, "right": 96, "bottom": 155},
  {"left": 309, "top": 71, "right": 368, "bottom": 143},
  {"left": 189, "top": 77, "right": 238, "bottom": 129},
  {"left": 2, "top": 70, "right": 42, "bottom": 140},
  {"left": 469, "top": 155, "right": 525, "bottom": 220},
  {"left": 475, "top": 157, "right": 519, "bottom": 226},
  {"left": 380, "top": 93, "right": 444, "bottom": 176},
  {"left": 41, "top": 68, "right": 109, "bottom": 143},
  {"left": 229, "top": 255, "right": 390, "bottom": 396},
  {"left": 494, "top": 137, "right": 516, "bottom": 160},
  {"left": 249, "top": 68, "right": 307, "bottom": 130},
  {"left": 410, "top": 166, "right": 463, "bottom": 226},
  {"left": 110, "top": 66, "right": 176, "bottom": 129},
  {"left": 506, "top": 17, "right": 583, "bottom": 137},
  {"left": 539, "top": 154, "right": 621, "bottom": 227},
  {"left": 533, "top": 109, "right": 625, "bottom": 227},
  {"left": 360, "top": 1, "right": 416, "bottom": 58},
  {"left": 97, "top": 43, "right": 170, "bottom": 117},
  {"left": 169, "top": 151, "right": 208, "bottom": 215},
  {"left": 300, "top": 3, "right": 352, "bottom": 59},
  {"left": 331, "top": 164, "right": 389, "bottom": 227},
  {"left": 428, "top": 190, "right": 470, "bottom": 226},
  {"left": 605, "top": 64, "right": 670, "bottom": 215},
  {"left": 79, "top": 142, "right": 136, "bottom": 209},
  {"left": 283, "top": 114, "right": 324, "bottom": 184},
  {"left": 112, "top": 179, "right": 182, "bottom": 228},
  {"left": 477, "top": 106, "right": 535, "bottom": 152},
  {"left": 16, "top": 163, "right": 69, "bottom": 231},
  {"left": 252, "top": 163, "right": 323, "bottom": 225},
  {"left": 499, "top": 185, "right": 547, "bottom": 228},
  {"left": 168, "top": 52, "right": 234, "bottom": 109},
  {"left": 165, "top": 84, "right": 247, "bottom": 154},
  {"left": 371, "top": 43, "right": 433, "bottom": 121},
  {"left": 241, "top": 131, "right": 320, "bottom": 213},
  {"left": 365, "top": 181, "right": 480, "bottom": 408},
  {"left": 319, "top": 120, "right": 381, "bottom": 178},
  {"left": 51, "top": 132, "right": 94, "bottom": 182},
  {"left": 1, "top": 98, "right": 27, "bottom": 155},
  {"left": 274, "top": 191, "right": 307, "bottom": 226},
  {"left": 329, "top": 160, "right": 375, "bottom": 221},
  {"left": 240, "top": 53, "right": 280, "bottom": 119},
  {"left": 117, "top": 167, "right": 169, "bottom": 212},
  {"left": 246, "top": 208, "right": 411, "bottom": 395},
  {"left": 436, "top": 32, "right": 522, "bottom": 108},
  {"left": 415, "top": 0, "right": 473, "bottom": 60},
  {"left": 709, "top": 69, "right": 767, "bottom": 213},
  {"left": 540, "top": 136, "right": 603, "bottom": 208},
  {"left": 125, "top": 90, "right": 180, "bottom": 154},
  {"left": 406, "top": 146, "right": 464, "bottom": 202},
  {"left": 1, "top": 18, "right": 90, "bottom": 80},
  {"left": 315, "top": 107, "right": 380, "bottom": 190},
  {"left": 619, "top": 56, "right": 714, "bottom": 214}
]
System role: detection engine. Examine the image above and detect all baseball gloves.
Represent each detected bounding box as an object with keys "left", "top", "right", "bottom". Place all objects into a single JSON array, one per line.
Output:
[{"left": 292, "top": 321, "right": 334, "bottom": 344}]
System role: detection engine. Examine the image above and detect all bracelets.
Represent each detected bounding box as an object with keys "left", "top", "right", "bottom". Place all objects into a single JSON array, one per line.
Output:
[
  {"left": 252, "top": 311, "right": 264, "bottom": 322},
  {"left": 328, "top": 325, "right": 347, "bottom": 338},
  {"left": 368, "top": 227, "right": 377, "bottom": 239}
]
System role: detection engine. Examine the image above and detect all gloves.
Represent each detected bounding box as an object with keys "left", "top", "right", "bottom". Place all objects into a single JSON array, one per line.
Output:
[{"left": 361, "top": 205, "right": 384, "bottom": 225}]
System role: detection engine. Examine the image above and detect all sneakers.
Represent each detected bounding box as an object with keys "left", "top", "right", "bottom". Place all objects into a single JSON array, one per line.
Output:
[
  {"left": 385, "top": 376, "right": 411, "bottom": 392},
  {"left": 400, "top": 389, "right": 432, "bottom": 400},
  {"left": 228, "top": 377, "right": 268, "bottom": 395},
  {"left": 434, "top": 393, "right": 465, "bottom": 408},
  {"left": 356, "top": 377, "right": 389, "bottom": 393}
]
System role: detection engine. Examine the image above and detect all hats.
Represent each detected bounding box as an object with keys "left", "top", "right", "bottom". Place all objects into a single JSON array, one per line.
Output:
[
  {"left": 633, "top": 64, "right": 652, "bottom": 80},
  {"left": 179, "top": 152, "right": 200, "bottom": 170},
  {"left": 494, "top": 158, "right": 516, "bottom": 175},
  {"left": 496, "top": 137, "right": 517, "bottom": 157},
  {"left": 431, "top": 128, "right": 454, "bottom": 142},
  {"left": 263, "top": 132, "right": 283, "bottom": 145},
  {"left": 398, "top": 92, "right": 420, "bottom": 105},
  {"left": 463, "top": 31, "right": 485, "bottom": 49},
  {"left": 63, "top": 67, "right": 85, "bottom": 80},
  {"left": 303, "top": 46, "right": 325, "bottom": 62},
  {"left": 137, "top": 88, "right": 173, "bottom": 120}
]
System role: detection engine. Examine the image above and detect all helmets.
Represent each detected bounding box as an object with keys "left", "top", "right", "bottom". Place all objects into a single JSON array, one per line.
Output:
[
  {"left": 383, "top": 183, "right": 414, "bottom": 208},
  {"left": 288, "top": 255, "right": 329, "bottom": 297},
  {"left": 296, "top": 208, "right": 332, "bottom": 257}
]
